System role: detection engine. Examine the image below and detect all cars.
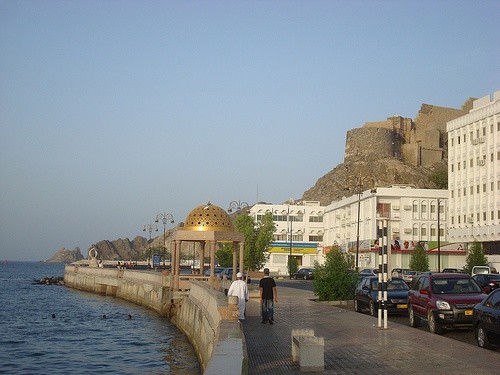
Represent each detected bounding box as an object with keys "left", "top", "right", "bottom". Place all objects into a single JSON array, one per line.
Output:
[
  {"left": 359, "top": 268, "right": 382, "bottom": 277},
  {"left": 203, "top": 266, "right": 226, "bottom": 277},
  {"left": 472, "top": 287, "right": 500, "bottom": 350},
  {"left": 354, "top": 275, "right": 411, "bottom": 318},
  {"left": 471, "top": 273, "right": 500, "bottom": 294},
  {"left": 219, "top": 267, "right": 251, "bottom": 284},
  {"left": 391, "top": 267, "right": 416, "bottom": 283},
  {"left": 292, "top": 268, "right": 316, "bottom": 280}
]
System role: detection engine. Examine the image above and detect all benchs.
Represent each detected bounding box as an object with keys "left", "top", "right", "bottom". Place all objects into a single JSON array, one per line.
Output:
[{"left": 291, "top": 329, "right": 324, "bottom": 372}]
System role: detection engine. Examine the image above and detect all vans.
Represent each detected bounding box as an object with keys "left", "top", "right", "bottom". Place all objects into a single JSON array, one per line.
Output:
[{"left": 471, "top": 266, "right": 491, "bottom": 277}]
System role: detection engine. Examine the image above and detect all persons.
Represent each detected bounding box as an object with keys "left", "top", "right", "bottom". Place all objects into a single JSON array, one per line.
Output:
[
  {"left": 259, "top": 268, "right": 278, "bottom": 325},
  {"left": 99, "top": 260, "right": 103, "bottom": 268},
  {"left": 227, "top": 272, "right": 249, "bottom": 328},
  {"left": 116, "top": 261, "right": 126, "bottom": 269}
]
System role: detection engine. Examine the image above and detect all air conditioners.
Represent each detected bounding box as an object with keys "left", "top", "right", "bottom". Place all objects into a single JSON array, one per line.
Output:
[
  {"left": 479, "top": 160, "right": 485, "bottom": 166},
  {"left": 467, "top": 217, "right": 474, "bottom": 222},
  {"left": 472, "top": 137, "right": 484, "bottom": 145}
]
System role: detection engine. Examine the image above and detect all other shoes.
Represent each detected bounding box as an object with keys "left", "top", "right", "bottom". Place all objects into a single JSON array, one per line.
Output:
[
  {"left": 262, "top": 320, "right": 267, "bottom": 323},
  {"left": 268, "top": 319, "right": 273, "bottom": 325}
]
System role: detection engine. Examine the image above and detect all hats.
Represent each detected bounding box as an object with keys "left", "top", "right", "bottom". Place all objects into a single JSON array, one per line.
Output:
[{"left": 237, "top": 272, "right": 242, "bottom": 277}]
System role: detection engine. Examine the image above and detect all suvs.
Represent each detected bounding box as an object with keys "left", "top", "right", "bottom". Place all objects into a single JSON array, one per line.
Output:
[{"left": 406, "top": 268, "right": 488, "bottom": 336}]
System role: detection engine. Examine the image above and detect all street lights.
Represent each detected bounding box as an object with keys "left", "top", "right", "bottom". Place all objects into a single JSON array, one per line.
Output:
[
  {"left": 154, "top": 209, "right": 175, "bottom": 270},
  {"left": 343, "top": 170, "right": 377, "bottom": 271},
  {"left": 142, "top": 221, "right": 159, "bottom": 271},
  {"left": 227, "top": 200, "right": 252, "bottom": 216}
]
